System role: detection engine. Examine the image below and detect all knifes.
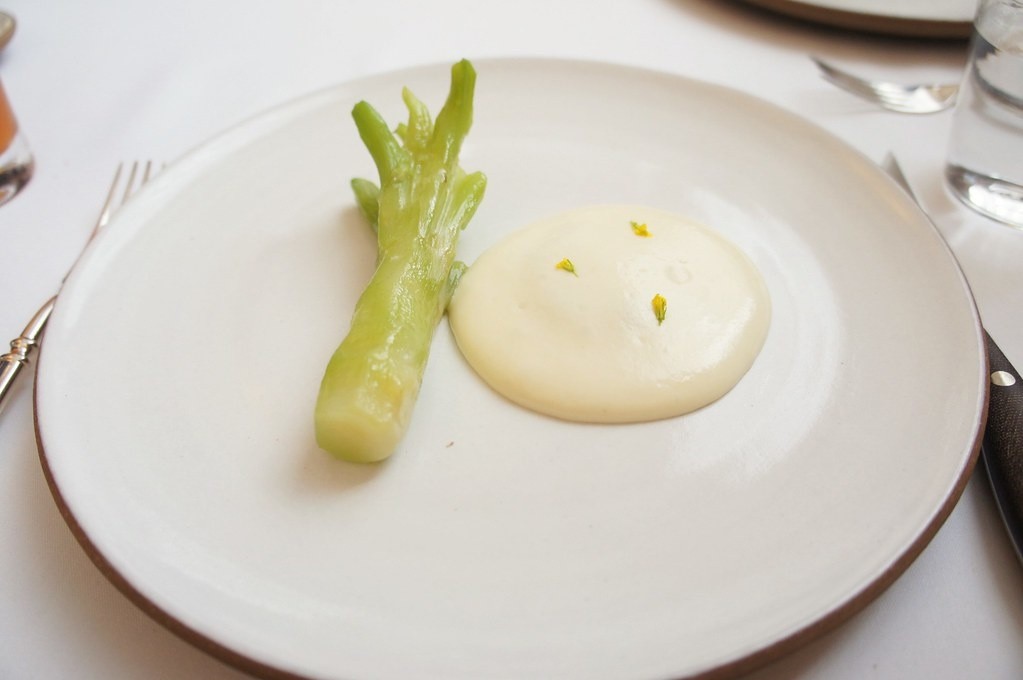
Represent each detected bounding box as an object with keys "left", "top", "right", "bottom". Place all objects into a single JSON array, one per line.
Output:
[
  {"left": 877, "top": 148, "right": 1023, "bottom": 577},
  {"left": 940, "top": 160, "right": 1023, "bottom": 233}
]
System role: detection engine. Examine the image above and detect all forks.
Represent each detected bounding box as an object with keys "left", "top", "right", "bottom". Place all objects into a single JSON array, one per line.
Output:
[
  {"left": 0, "top": 158, "right": 167, "bottom": 419},
  {"left": 811, "top": 51, "right": 963, "bottom": 116}
]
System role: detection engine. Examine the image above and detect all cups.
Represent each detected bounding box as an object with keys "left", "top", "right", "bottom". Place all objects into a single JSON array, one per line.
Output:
[
  {"left": 941, "top": 1, "right": 1023, "bottom": 233},
  {"left": 0, "top": 65, "right": 38, "bottom": 208}
]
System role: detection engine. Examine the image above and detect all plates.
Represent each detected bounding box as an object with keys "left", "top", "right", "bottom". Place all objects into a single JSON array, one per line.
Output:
[
  {"left": 33, "top": 53, "right": 992, "bottom": 679},
  {"left": 730, "top": 0, "right": 987, "bottom": 42}
]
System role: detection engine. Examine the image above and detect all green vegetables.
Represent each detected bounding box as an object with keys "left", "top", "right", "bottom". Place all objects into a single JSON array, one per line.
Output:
[{"left": 316, "top": 59, "right": 487, "bottom": 463}]
